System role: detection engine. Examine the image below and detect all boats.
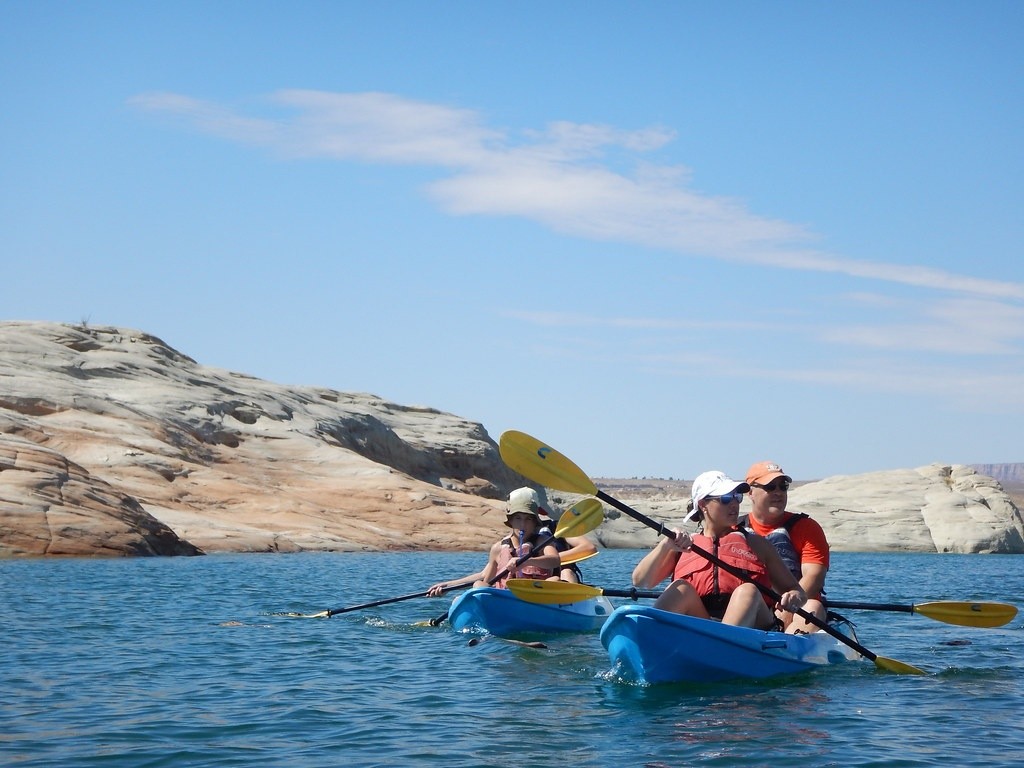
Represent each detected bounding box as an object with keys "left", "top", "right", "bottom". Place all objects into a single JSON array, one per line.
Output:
[
  {"left": 449, "top": 587, "right": 615, "bottom": 637},
  {"left": 600, "top": 605, "right": 863, "bottom": 686}
]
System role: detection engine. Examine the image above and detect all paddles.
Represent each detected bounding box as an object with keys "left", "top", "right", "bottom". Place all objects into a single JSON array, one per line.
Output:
[
  {"left": 499, "top": 576, "right": 1019, "bottom": 631},
  {"left": 499, "top": 429, "right": 928, "bottom": 676},
  {"left": 411, "top": 498, "right": 604, "bottom": 627},
  {"left": 311, "top": 550, "right": 601, "bottom": 619}
]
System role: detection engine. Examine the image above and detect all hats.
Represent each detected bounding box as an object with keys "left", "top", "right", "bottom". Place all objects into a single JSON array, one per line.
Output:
[
  {"left": 683, "top": 471, "right": 751, "bottom": 524},
  {"left": 506, "top": 487, "right": 548, "bottom": 515},
  {"left": 503, "top": 497, "right": 543, "bottom": 527},
  {"left": 745, "top": 461, "right": 792, "bottom": 485}
]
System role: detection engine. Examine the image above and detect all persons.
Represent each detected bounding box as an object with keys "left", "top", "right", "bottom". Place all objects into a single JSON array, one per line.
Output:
[
  {"left": 732, "top": 461, "right": 830, "bottom": 635},
  {"left": 632, "top": 471, "right": 807, "bottom": 629},
  {"left": 426, "top": 486, "right": 598, "bottom": 597}
]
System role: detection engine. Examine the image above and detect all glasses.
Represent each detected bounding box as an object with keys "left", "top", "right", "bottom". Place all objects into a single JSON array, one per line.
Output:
[
  {"left": 750, "top": 483, "right": 789, "bottom": 492},
  {"left": 703, "top": 492, "right": 744, "bottom": 504}
]
